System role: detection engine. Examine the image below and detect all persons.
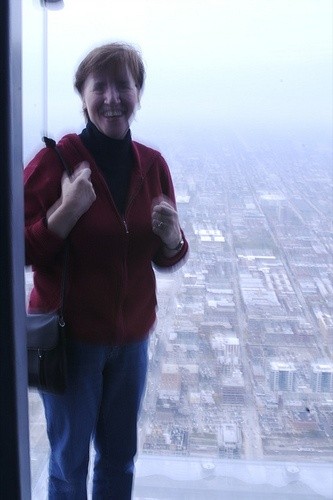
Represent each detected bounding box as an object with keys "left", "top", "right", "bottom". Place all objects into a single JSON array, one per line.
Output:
[{"left": 23, "top": 44, "right": 188, "bottom": 500}]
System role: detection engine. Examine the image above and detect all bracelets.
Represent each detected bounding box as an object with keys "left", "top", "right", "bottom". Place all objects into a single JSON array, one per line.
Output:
[{"left": 165, "top": 240, "right": 184, "bottom": 250}]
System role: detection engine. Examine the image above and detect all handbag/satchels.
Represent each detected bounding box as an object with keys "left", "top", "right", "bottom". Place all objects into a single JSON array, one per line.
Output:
[{"left": 25, "top": 308, "right": 82, "bottom": 394}]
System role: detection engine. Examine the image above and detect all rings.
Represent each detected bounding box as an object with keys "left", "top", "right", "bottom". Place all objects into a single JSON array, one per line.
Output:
[{"left": 157, "top": 221, "right": 163, "bottom": 227}]
